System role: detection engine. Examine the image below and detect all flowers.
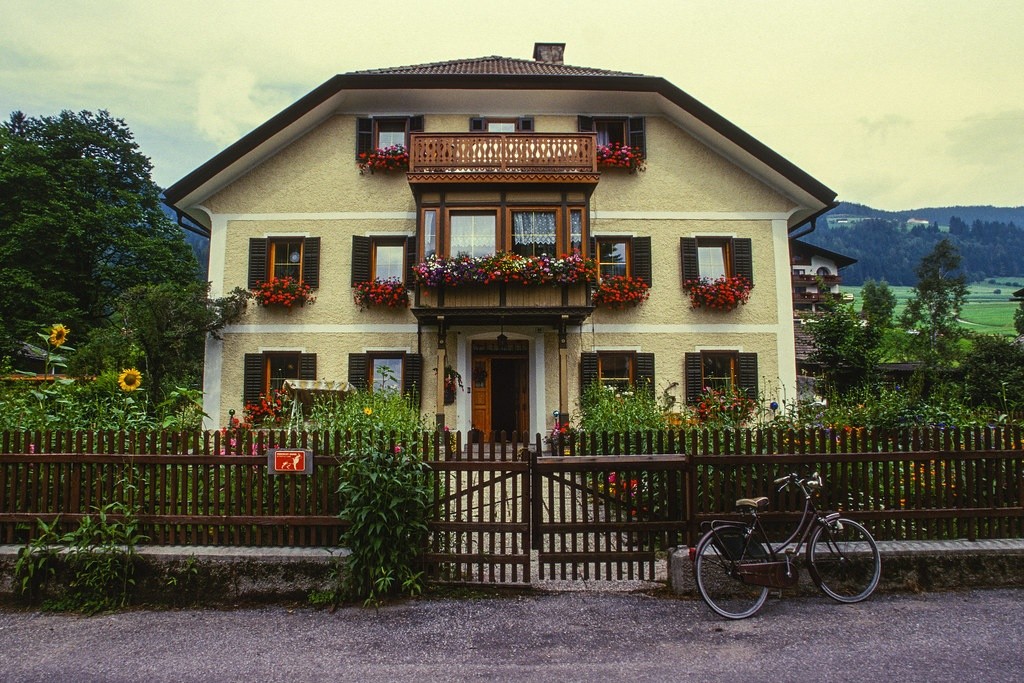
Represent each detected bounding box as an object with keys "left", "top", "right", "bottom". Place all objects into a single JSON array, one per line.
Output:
[
  {"left": 412, "top": 252, "right": 599, "bottom": 292},
  {"left": 695, "top": 387, "right": 759, "bottom": 423},
  {"left": 353, "top": 276, "right": 414, "bottom": 310},
  {"left": 441, "top": 365, "right": 465, "bottom": 405},
  {"left": 251, "top": 275, "right": 317, "bottom": 310},
  {"left": 359, "top": 144, "right": 408, "bottom": 174},
  {"left": 549, "top": 423, "right": 574, "bottom": 446},
  {"left": 592, "top": 140, "right": 647, "bottom": 174},
  {"left": 591, "top": 276, "right": 651, "bottom": 311},
  {"left": 684, "top": 273, "right": 756, "bottom": 311}
]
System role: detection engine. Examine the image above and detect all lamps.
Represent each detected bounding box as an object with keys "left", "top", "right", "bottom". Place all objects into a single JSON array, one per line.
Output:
[{"left": 496, "top": 321, "right": 509, "bottom": 350}]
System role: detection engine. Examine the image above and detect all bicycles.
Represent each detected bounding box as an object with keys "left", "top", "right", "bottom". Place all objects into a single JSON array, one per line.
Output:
[{"left": 689, "top": 472, "right": 882, "bottom": 620}]
